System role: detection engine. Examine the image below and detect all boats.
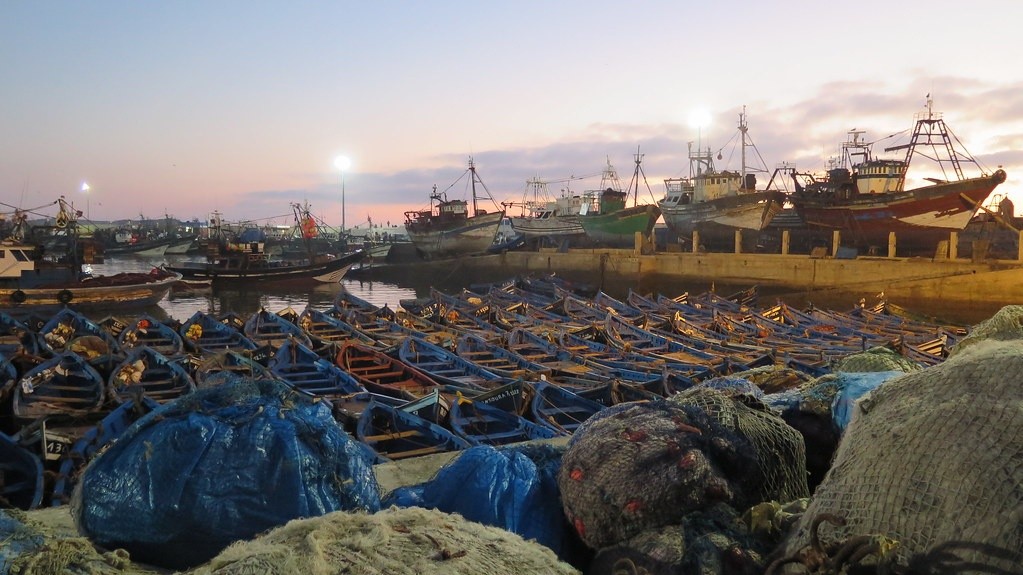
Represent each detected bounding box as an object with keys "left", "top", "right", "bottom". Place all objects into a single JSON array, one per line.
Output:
[
  {"left": 655, "top": 105, "right": 786, "bottom": 248},
  {"left": 574, "top": 143, "right": 663, "bottom": 248},
  {"left": 0, "top": 194, "right": 974, "bottom": 514},
  {"left": 402, "top": 155, "right": 506, "bottom": 260},
  {"left": 785, "top": 91, "right": 1008, "bottom": 256},
  {"left": 500, "top": 169, "right": 604, "bottom": 253}
]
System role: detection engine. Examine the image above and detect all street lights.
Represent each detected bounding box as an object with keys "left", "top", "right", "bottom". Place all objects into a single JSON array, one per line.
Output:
[
  {"left": 335, "top": 155, "right": 353, "bottom": 234},
  {"left": 80, "top": 182, "right": 90, "bottom": 235}
]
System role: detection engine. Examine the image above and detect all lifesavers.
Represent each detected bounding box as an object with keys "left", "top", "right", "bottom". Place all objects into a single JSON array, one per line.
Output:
[
  {"left": 57, "top": 289, "right": 74, "bottom": 303},
  {"left": 12, "top": 291, "right": 27, "bottom": 302}
]
